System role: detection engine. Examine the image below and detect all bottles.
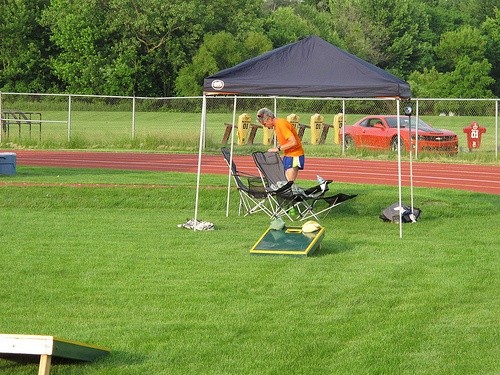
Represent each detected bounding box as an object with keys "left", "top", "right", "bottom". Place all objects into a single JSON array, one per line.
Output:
[{"left": 316, "top": 175, "right": 326, "bottom": 191}]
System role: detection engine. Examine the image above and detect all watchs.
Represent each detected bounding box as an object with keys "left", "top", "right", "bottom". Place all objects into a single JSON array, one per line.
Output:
[{"left": 277, "top": 146, "right": 281, "bottom": 152}]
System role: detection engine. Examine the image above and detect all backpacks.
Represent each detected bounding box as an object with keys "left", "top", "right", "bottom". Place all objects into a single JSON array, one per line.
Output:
[{"left": 379, "top": 202, "right": 422, "bottom": 224}]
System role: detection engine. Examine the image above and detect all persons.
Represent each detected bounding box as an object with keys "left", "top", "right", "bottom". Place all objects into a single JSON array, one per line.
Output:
[{"left": 256, "top": 106, "right": 305, "bottom": 184}]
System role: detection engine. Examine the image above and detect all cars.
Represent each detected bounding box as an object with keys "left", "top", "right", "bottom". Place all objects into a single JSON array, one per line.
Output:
[{"left": 338, "top": 114, "right": 459, "bottom": 156}]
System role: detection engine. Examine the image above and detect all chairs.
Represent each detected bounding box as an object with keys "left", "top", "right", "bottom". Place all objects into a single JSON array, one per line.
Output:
[
  {"left": 243, "top": 151, "right": 359, "bottom": 223},
  {"left": 220, "top": 146, "right": 333, "bottom": 217}
]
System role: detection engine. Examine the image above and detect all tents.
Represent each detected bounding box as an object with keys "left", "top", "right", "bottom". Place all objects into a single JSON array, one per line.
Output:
[{"left": 194, "top": 36, "right": 414, "bottom": 239}]
